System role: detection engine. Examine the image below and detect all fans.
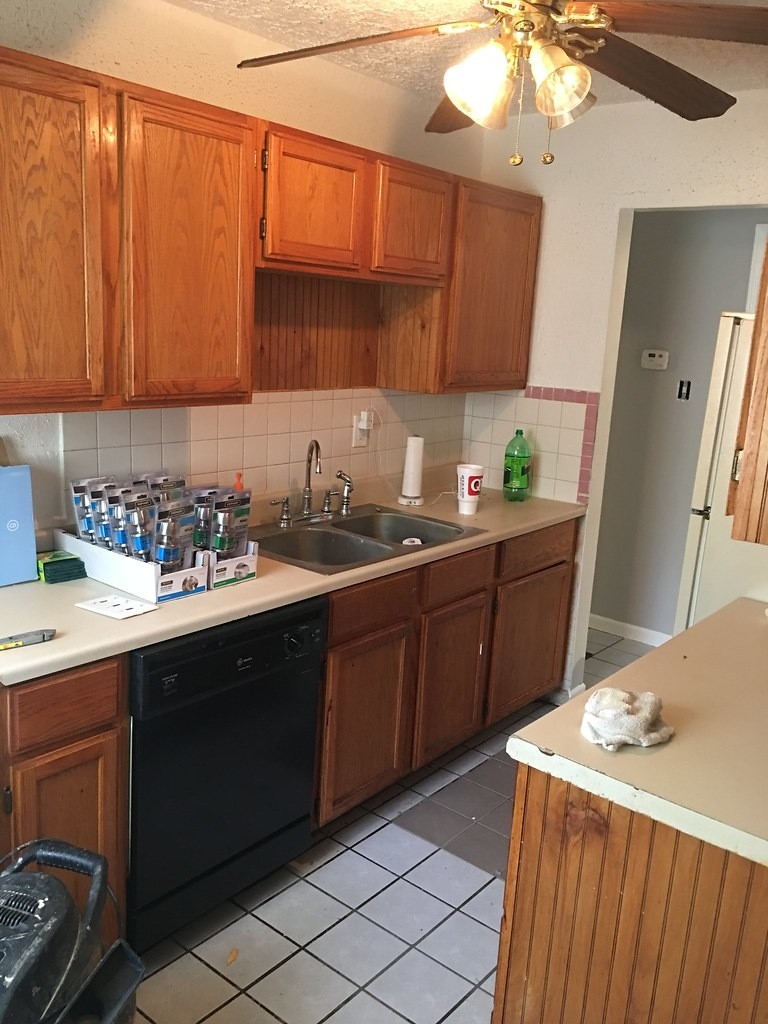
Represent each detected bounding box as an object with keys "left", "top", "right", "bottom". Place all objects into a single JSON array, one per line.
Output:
[{"left": 235, "top": 0, "right": 767, "bottom": 134}]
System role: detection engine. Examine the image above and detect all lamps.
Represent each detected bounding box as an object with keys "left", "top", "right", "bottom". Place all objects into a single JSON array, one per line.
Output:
[{"left": 443, "top": 0, "right": 598, "bottom": 164}]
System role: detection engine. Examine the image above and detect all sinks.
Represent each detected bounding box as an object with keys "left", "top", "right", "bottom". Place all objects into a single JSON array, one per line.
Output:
[
  {"left": 248, "top": 521, "right": 436, "bottom": 575},
  {"left": 323, "top": 503, "right": 489, "bottom": 546}
]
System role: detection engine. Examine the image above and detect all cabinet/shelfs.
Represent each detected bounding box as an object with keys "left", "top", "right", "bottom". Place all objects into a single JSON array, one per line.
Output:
[
  {"left": 726, "top": 236, "right": 768, "bottom": 546},
  {"left": 316, "top": 565, "right": 422, "bottom": 828},
  {"left": 0, "top": 654, "right": 129, "bottom": 955},
  {"left": 1, "top": 46, "right": 258, "bottom": 414},
  {"left": 412, "top": 542, "right": 499, "bottom": 772},
  {"left": 484, "top": 518, "right": 580, "bottom": 727},
  {"left": 376, "top": 175, "right": 544, "bottom": 395},
  {"left": 254, "top": 119, "right": 455, "bottom": 290}
]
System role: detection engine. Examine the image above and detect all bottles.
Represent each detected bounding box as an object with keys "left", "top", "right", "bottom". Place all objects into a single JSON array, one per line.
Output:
[{"left": 503, "top": 430, "right": 531, "bottom": 502}]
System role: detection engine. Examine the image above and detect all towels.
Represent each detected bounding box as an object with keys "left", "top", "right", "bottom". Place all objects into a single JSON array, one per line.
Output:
[{"left": 579, "top": 688, "right": 676, "bottom": 753}]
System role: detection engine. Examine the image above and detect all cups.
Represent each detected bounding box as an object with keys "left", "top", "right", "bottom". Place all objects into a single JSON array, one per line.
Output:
[{"left": 456, "top": 464, "right": 484, "bottom": 515}]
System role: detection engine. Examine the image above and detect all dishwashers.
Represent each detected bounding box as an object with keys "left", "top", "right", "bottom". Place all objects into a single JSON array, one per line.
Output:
[{"left": 126, "top": 593, "right": 332, "bottom": 956}]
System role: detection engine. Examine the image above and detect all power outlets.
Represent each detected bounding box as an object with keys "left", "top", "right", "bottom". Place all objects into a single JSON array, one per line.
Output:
[{"left": 352, "top": 416, "right": 368, "bottom": 448}]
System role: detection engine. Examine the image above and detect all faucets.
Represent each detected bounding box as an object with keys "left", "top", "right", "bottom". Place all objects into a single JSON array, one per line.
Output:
[{"left": 301, "top": 440, "right": 323, "bottom": 515}]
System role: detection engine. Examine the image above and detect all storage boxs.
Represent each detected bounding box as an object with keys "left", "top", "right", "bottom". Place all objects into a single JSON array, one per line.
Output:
[{"left": 54, "top": 525, "right": 260, "bottom": 604}]
